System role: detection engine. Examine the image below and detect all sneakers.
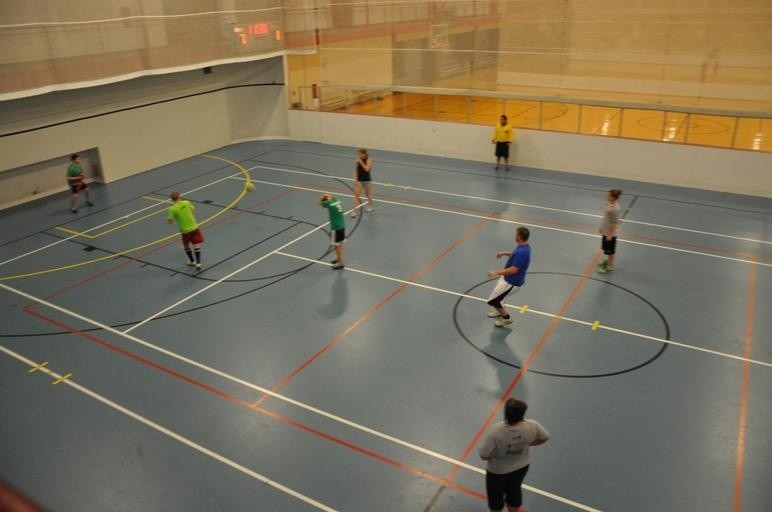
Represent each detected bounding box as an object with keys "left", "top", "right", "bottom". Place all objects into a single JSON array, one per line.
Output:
[
  {"left": 486, "top": 309, "right": 504, "bottom": 318},
  {"left": 364, "top": 205, "right": 375, "bottom": 213},
  {"left": 351, "top": 211, "right": 357, "bottom": 218},
  {"left": 493, "top": 316, "right": 514, "bottom": 328},
  {"left": 597, "top": 259, "right": 609, "bottom": 266},
  {"left": 70, "top": 200, "right": 93, "bottom": 214},
  {"left": 187, "top": 259, "right": 202, "bottom": 272},
  {"left": 597, "top": 264, "right": 614, "bottom": 274},
  {"left": 331, "top": 256, "right": 345, "bottom": 270}
]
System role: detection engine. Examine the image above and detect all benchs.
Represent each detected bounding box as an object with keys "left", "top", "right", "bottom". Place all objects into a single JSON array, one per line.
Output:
[
  {"left": 320, "top": 92, "right": 350, "bottom": 112},
  {"left": 355, "top": 87, "right": 378, "bottom": 100}
]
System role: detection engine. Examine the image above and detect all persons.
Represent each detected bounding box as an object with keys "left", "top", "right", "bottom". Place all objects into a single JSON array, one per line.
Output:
[
  {"left": 486, "top": 227, "right": 531, "bottom": 328},
  {"left": 596, "top": 188, "right": 623, "bottom": 274},
  {"left": 478, "top": 397, "right": 550, "bottom": 512},
  {"left": 351, "top": 148, "right": 374, "bottom": 218},
  {"left": 317, "top": 195, "right": 345, "bottom": 269},
  {"left": 490, "top": 115, "right": 514, "bottom": 171},
  {"left": 65, "top": 154, "right": 94, "bottom": 214},
  {"left": 167, "top": 192, "right": 205, "bottom": 269}
]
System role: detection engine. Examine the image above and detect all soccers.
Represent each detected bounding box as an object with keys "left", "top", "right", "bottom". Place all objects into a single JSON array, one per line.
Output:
[{"left": 245, "top": 182, "right": 254, "bottom": 190}]
